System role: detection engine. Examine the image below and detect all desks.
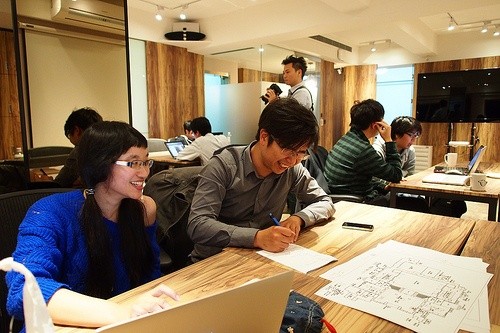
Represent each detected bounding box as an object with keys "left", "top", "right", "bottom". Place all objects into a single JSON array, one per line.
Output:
[
  {"left": 5, "top": 158, "right": 24, "bottom": 167},
  {"left": 58, "top": 201, "right": 500, "bottom": 333},
  {"left": 389, "top": 160, "right": 500, "bottom": 222},
  {"left": 148, "top": 151, "right": 200, "bottom": 180}
]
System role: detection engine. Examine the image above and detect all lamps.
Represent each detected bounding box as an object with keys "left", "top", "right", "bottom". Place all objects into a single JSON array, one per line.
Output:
[
  {"left": 180, "top": 6, "right": 190, "bottom": 20},
  {"left": 493, "top": 27, "right": 500, "bottom": 37},
  {"left": 480, "top": 22, "right": 488, "bottom": 33},
  {"left": 369, "top": 42, "right": 377, "bottom": 52},
  {"left": 156, "top": 7, "right": 164, "bottom": 20},
  {"left": 447, "top": 18, "right": 455, "bottom": 31}
]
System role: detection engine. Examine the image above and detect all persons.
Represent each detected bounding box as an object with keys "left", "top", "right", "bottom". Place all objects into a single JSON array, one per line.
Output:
[
  {"left": 175, "top": 120, "right": 195, "bottom": 148},
  {"left": 185, "top": 98, "right": 335, "bottom": 265},
  {"left": 264, "top": 55, "right": 314, "bottom": 215},
  {"left": 4, "top": 118, "right": 180, "bottom": 333},
  {"left": 373, "top": 115, "right": 425, "bottom": 211},
  {"left": 324, "top": 99, "right": 404, "bottom": 208},
  {"left": 50, "top": 107, "right": 106, "bottom": 183},
  {"left": 176, "top": 116, "right": 232, "bottom": 168}
]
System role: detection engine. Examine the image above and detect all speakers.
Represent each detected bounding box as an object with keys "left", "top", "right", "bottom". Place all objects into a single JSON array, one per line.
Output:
[{"left": 164, "top": 22, "right": 206, "bottom": 41}]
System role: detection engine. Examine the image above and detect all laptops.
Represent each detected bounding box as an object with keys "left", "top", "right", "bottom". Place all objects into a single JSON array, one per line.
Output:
[
  {"left": 434, "top": 144, "right": 485, "bottom": 175},
  {"left": 91, "top": 269, "right": 294, "bottom": 332},
  {"left": 422, "top": 147, "right": 486, "bottom": 186},
  {"left": 164, "top": 141, "right": 185, "bottom": 159}
]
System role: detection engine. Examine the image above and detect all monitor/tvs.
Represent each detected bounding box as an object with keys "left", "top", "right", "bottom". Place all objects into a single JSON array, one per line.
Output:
[{"left": 415, "top": 67, "right": 500, "bottom": 123}]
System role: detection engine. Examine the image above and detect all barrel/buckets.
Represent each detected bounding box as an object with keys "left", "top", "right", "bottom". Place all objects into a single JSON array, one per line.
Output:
[{"left": 448, "top": 144, "right": 468, "bottom": 164}]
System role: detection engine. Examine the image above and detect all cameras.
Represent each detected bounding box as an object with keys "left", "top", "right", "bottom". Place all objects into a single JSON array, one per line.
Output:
[{"left": 260, "top": 84, "right": 282, "bottom": 105}]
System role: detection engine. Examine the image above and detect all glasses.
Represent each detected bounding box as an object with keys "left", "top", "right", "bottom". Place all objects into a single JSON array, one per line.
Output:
[
  {"left": 405, "top": 132, "right": 420, "bottom": 139},
  {"left": 114, "top": 159, "right": 154, "bottom": 169},
  {"left": 270, "top": 134, "right": 311, "bottom": 160}
]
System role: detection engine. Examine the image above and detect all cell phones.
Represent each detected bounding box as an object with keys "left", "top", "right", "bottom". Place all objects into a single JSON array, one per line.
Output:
[{"left": 342, "top": 222, "right": 374, "bottom": 231}]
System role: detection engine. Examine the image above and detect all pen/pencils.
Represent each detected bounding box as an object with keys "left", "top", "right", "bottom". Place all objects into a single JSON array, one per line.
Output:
[
  {"left": 39, "top": 167, "right": 48, "bottom": 177},
  {"left": 268, "top": 212, "right": 283, "bottom": 228}
]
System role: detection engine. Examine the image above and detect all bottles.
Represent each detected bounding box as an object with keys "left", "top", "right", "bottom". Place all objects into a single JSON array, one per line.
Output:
[
  {"left": 227, "top": 132, "right": 231, "bottom": 143},
  {"left": 14, "top": 147, "right": 24, "bottom": 160}
]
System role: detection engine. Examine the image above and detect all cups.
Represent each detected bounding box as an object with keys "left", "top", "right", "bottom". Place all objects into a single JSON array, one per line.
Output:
[
  {"left": 465, "top": 173, "right": 486, "bottom": 191},
  {"left": 444, "top": 152, "right": 458, "bottom": 167}
]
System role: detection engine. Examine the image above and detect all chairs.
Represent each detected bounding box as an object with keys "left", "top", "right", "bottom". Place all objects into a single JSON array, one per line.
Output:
[
  {"left": 146, "top": 139, "right": 169, "bottom": 152},
  {"left": 0, "top": 189, "right": 78, "bottom": 333},
  {"left": 300, "top": 146, "right": 364, "bottom": 204},
  {"left": 143, "top": 167, "right": 206, "bottom": 276}
]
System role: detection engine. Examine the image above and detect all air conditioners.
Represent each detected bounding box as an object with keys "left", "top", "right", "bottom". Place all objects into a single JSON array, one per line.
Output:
[{"left": 51, "top": 0, "right": 126, "bottom": 36}]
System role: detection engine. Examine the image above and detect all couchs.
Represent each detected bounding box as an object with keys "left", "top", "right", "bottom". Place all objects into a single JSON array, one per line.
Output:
[{"left": 30, "top": 146, "right": 74, "bottom": 167}]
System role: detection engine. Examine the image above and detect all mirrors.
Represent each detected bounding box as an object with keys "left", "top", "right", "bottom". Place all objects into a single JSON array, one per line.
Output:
[{"left": 16, "top": 0, "right": 130, "bottom": 182}]
